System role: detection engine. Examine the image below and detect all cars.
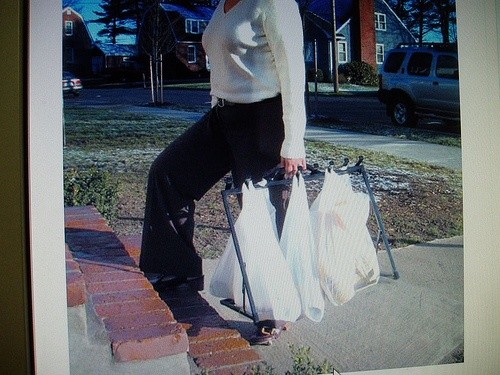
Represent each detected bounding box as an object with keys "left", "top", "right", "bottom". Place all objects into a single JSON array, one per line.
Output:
[{"left": 62, "top": 70, "right": 83, "bottom": 97}]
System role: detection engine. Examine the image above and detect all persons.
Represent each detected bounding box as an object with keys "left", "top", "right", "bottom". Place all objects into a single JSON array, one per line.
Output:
[{"left": 143, "top": 1, "right": 306, "bottom": 345}]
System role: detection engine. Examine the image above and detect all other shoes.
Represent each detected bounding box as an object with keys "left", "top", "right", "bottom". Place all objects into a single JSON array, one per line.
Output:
[
  {"left": 249, "top": 325, "right": 284, "bottom": 344},
  {"left": 150, "top": 274, "right": 204, "bottom": 289}
]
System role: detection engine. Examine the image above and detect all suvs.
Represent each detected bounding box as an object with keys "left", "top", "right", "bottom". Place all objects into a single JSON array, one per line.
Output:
[{"left": 377, "top": 42, "right": 461, "bottom": 134}]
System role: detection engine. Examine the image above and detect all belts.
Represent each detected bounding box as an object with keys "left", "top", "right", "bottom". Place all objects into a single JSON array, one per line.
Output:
[{"left": 216, "top": 98, "right": 236, "bottom": 108}]
